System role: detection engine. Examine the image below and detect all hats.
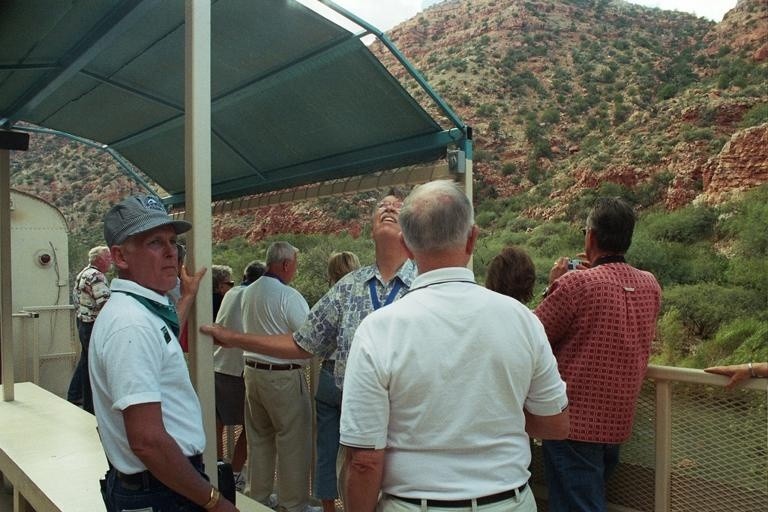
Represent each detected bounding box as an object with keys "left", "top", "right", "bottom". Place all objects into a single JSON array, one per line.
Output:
[{"left": 103, "top": 193, "right": 193, "bottom": 249}]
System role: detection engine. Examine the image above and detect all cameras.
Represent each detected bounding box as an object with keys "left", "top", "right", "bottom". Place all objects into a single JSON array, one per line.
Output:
[{"left": 568, "top": 260, "right": 583, "bottom": 270}]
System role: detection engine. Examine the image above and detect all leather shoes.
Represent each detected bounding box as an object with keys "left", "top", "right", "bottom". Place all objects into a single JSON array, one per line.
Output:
[{"left": 68, "top": 398, "right": 84, "bottom": 405}]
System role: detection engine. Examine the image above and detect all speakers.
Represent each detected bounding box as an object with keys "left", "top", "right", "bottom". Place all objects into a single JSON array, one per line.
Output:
[{"left": 1, "top": 130, "right": 30, "bottom": 151}]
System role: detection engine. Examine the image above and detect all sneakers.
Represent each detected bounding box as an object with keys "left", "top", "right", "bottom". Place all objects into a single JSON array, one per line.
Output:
[
  {"left": 267, "top": 493, "right": 278, "bottom": 508},
  {"left": 305, "top": 505, "right": 322, "bottom": 512},
  {"left": 233, "top": 473, "right": 247, "bottom": 491}
]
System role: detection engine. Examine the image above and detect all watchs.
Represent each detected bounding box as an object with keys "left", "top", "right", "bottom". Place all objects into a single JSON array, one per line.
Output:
[{"left": 203, "top": 485, "right": 221, "bottom": 508}]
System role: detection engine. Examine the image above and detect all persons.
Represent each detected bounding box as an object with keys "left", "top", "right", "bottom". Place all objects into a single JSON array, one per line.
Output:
[
  {"left": 214, "top": 260, "right": 267, "bottom": 492},
  {"left": 213, "top": 264, "right": 234, "bottom": 323},
  {"left": 337, "top": 180, "right": 570, "bottom": 511},
  {"left": 703, "top": 361, "right": 768, "bottom": 390},
  {"left": 67, "top": 245, "right": 112, "bottom": 415},
  {"left": 241, "top": 241, "right": 326, "bottom": 511},
  {"left": 486, "top": 245, "right": 535, "bottom": 304},
  {"left": 534, "top": 197, "right": 663, "bottom": 512},
  {"left": 314, "top": 251, "right": 361, "bottom": 511},
  {"left": 200, "top": 187, "right": 418, "bottom": 511},
  {"left": 169, "top": 243, "right": 186, "bottom": 302},
  {"left": 87, "top": 192, "right": 242, "bottom": 512}
]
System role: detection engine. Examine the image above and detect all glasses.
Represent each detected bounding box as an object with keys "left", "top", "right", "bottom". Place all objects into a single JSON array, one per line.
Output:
[
  {"left": 223, "top": 281, "right": 234, "bottom": 288},
  {"left": 373, "top": 199, "right": 406, "bottom": 210},
  {"left": 581, "top": 226, "right": 590, "bottom": 235}
]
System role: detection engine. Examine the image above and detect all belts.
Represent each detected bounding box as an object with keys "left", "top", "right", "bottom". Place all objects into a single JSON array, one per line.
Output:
[
  {"left": 391, "top": 481, "right": 529, "bottom": 509},
  {"left": 244, "top": 357, "right": 302, "bottom": 371},
  {"left": 110, "top": 454, "right": 204, "bottom": 485}
]
